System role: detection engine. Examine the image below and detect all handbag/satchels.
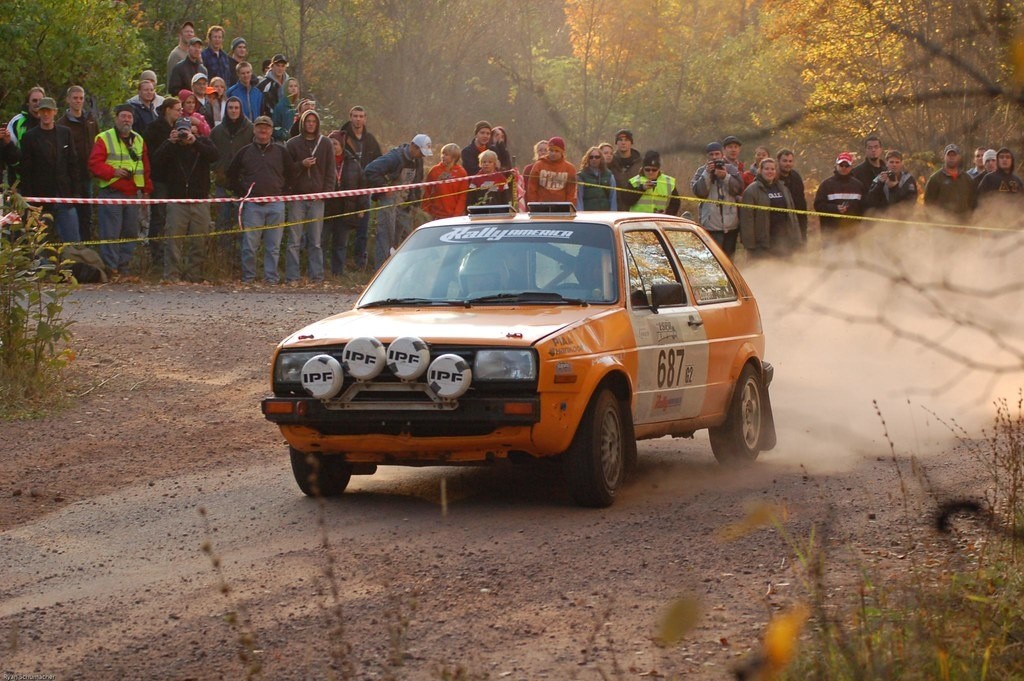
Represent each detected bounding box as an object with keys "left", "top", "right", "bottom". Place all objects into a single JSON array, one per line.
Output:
[{"left": 55, "top": 243, "right": 108, "bottom": 283}]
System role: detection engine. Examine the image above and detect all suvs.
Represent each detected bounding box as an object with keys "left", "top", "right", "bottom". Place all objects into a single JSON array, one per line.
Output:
[{"left": 262, "top": 203, "right": 775, "bottom": 509}]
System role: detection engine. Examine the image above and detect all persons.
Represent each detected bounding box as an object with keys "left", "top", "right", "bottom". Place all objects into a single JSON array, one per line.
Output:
[
  {"left": 689, "top": 142, "right": 744, "bottom": 264},
  {"left": 739, "top": 146, "right": 803, "bottom": 264},
  {"left": 923, "top": 143, "right": 977, "bottom": 222},
  {"left": 521, "top": 137, "right": 576, "bottom": 212},
  {"left": 85, "top": 104, "right": 154, "bottom": 282},
  {"left": 207, "top": 97, "right": 294, "bottom": 289},
  {"left": 459, "top": 247, "right": 510, "bottom": 299},
  {"left": 575, "top": 142, "right": 623, "bottom": 211},
  {"left": 461, "top": 120, "right": 514, "bottom": 214},
  {"left": 865, "top": 149, "right": 917, "bottom": 219},
  {"left": 421, "top": 142, "right": 468, "bottom": 223},
  {"left": 966, "top": 147, "right": 1024, "bottom": 198},
  {"left": 776, "top": 147, "right": 807, "bottom": 247},
  {"left": 167, "top": 21, "right": 289, "bottom": 121},
  {"left": 364, "top": 133, "right": 434, "bottom": 277},
  {"left": 721, "top": 136, "right": 746, "bottom": 174},
  {"left": 283, "top": 98, "right": 335, "bottom": 285},
  {"left": 143, "top": 90, "right": 220, "bottom": 283},
  {"left": 612, "top": 127, "right": 681, "bottom": 216},
  {"left": 814, "top": 152, "right": 867, "bottom": 242},
  {"left": 124, "top": 70, "right": 167, "bottom": 246},
  {"left": 0, "top": 84, "right": 101, "bottom": 286},
  {"left": 575, "top": 243, "right": 615, "bottom": 296},
  {"left": 326, "top": 105, "right": 382, "bottom": 276},
  {"left": 850, "top": 135, "right": 888, "bottom": 200}
]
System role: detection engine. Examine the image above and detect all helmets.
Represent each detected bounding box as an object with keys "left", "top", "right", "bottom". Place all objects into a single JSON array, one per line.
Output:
[{"left": 459, "top": 247, "right": 510, "bottom": 295}]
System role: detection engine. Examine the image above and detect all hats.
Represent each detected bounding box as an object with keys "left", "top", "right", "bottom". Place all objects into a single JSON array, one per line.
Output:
[
  {"left": 112, "top": 105, "right": 135, "bottom": 116},
  {"left": 39, "top": 97, "right": 56, "bottom": 110},
  {"left": 474, "top": 121, "right": 491, "bottom": 135},
  {"left": 327, "top": 130, "right": 345, "bottom": 147},
  {"left": 548, "top": 137, "right": 565, "bottom": 151},
  {"left": 643, "top": 151, "right": 661, "bottom": 169},
  {"left": 983, "top": 149, "right": 997, "bottom": 165},
  {"left": 836, "top": 152, "right": 853, "bottom": 166},
  {"left": 180, "top": 21, "right": 249, "bottom": 101},
  {"left": 723, "top": 135, "right": 741, "bottom": 148},
  {"left": 615, "top": 129, "right": 633, "bottom": 145},
  {"left": 945, "top": 144, "right": 960, "bottom": 155},
  {"left": 255, "top": 116, "right": 273, "bottom": 126},
  {"left": 141, "top": 71, "right": 156, "bottom": 83},
  {"left": 413, "top": 134, "right": 433, "bottom": 157},
  {"left": 272, "top": 54, "right": 287, "bottom": 64},
  {"left": 174, "top": 117, "right": 191, "bottom": 131},
  {"left": 707, "top": 142, "right": 723, "bottom": 153}
]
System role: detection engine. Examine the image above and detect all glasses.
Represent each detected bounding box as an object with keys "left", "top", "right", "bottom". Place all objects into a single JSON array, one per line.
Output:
[
  {"left": 590, "top": 155, "right": 601, "bottom": 159},
  {"left": 643, "top": 168, "right": 659, "bottom": 171},
  {"left": 462, "top": 273, "right": 500, "bottom": 292},
  {"left": 172, "top": 108, "right": 183, "bottom": 111}
]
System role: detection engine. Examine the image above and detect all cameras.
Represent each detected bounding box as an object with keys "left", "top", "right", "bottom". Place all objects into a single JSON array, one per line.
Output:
[
  {"left": 178, "top": 130, "right": 188, "bottom": 139},
  {"left": 843, "top": 201, "right": 850, "bottom": 209},
  {"left": 713, "top": 159, "right": 725, "bottom": 170},
  {"left": 650, "top": 181, "right": 658, "bottom": 186},
  {"left": 887, "top": 170, "right": 896, "bottom": 181}
]
